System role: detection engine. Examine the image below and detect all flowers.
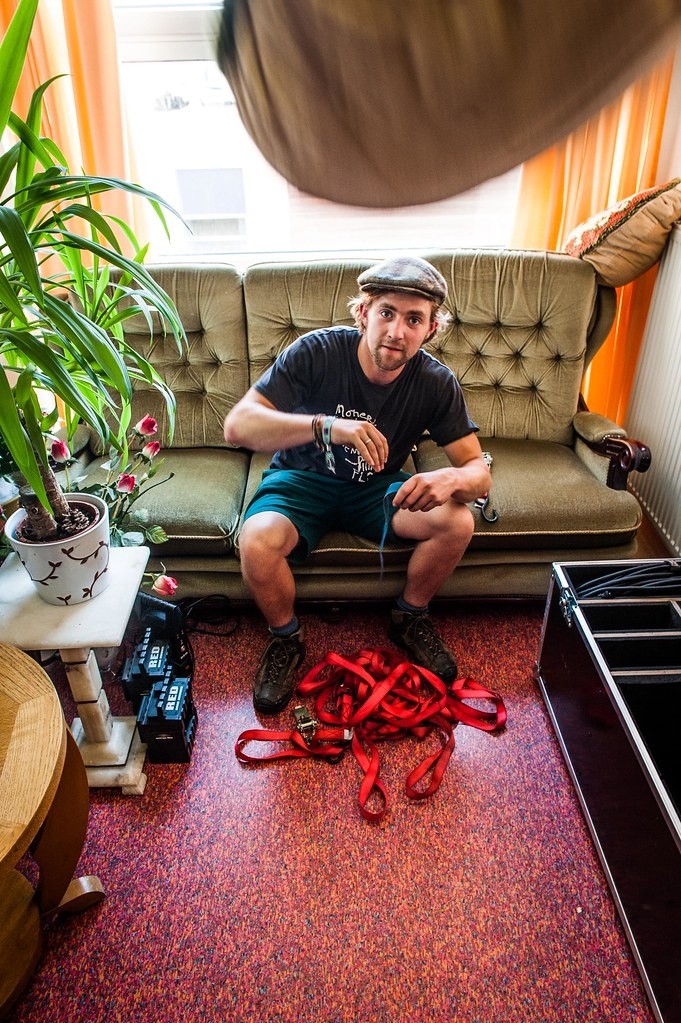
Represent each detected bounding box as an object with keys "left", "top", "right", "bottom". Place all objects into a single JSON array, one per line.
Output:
[{"left": 40, "top": 417, "right": 177, "bottom": 599}]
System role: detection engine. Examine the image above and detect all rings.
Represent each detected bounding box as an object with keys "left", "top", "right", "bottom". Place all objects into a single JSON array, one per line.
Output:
[{"left": 366, "top": 439, "right": 371, "bottom": 444}]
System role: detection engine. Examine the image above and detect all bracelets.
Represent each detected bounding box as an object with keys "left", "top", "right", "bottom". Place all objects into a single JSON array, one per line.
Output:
[
  {"left": 312, "top": 414, "right": 324, "bottom": 449},
  {"left": 322, "top": 415, "right": 336, "bottom": 452}
]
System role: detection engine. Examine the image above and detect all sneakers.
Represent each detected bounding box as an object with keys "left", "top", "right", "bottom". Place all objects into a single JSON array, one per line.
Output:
[
  {"left": 390, "top": 597, "right": 456, "bottom": 679},
  {"left": 253, "top": 625, "right": 306, "bottom": 712}
]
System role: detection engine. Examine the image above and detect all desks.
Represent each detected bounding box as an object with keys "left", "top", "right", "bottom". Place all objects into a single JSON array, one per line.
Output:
[
  {"left": 0, "top": 547, "right": 147, "bottom": 796},
  {"left": 0, "top": 643, "right": 105, "bottom": 1015}
]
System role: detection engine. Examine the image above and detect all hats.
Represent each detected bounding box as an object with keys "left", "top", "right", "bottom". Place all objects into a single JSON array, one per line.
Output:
[{"left": 356, "top": 257, "right": 447, "bottom": 305}]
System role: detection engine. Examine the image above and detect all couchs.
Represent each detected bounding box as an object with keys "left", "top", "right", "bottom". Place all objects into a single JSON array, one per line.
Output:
[{"left": 73, "top": 247, "right": 651, "bottom": 627}]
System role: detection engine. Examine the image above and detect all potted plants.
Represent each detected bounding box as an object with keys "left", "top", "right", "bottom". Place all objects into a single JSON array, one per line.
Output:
[{"left": 0, "top": 1, "right": 189, "bottom": 606}]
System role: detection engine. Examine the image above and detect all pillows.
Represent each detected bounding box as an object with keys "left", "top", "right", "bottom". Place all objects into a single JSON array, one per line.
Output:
[{"left": 562, "top": 178, "right": 681, "bottom": 287}]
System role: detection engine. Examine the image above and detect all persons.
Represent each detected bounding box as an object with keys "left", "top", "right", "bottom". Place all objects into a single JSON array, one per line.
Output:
[{"left": 222, "top": 256, "right": 493, "bottom": 711}]
